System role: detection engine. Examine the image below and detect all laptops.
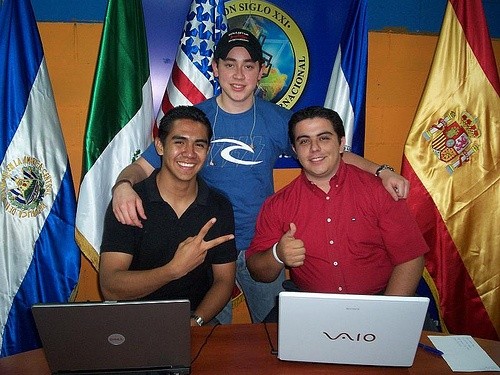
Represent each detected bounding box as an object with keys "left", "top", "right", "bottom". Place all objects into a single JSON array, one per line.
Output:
[
  {"left": 30, "top": 298, "right": 191, "bottom": 375},
  {"left": 277, "top": 291, "right": 431, "bottom": 366}
]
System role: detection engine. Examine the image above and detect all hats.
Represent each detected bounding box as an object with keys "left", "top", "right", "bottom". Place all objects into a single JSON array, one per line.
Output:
[{"left": 214, "top": 28, "right": 263, "bottom": 63}]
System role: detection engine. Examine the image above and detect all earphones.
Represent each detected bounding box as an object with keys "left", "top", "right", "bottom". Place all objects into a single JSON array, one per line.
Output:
[{"left": 209, "top": 161, "right": 214, "bottom": 165}]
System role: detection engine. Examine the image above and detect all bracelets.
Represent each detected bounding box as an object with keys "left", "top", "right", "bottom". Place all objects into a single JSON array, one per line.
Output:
[
  {"left": 112, "top": 179, "right": 133, "bottom": 191},
  {"left": 190, "top": 314, "right": 205, "bottom": 326},
  {"left": 375, "top": 164, "right": 396, "bottom": 177},
  {"left": 272, "top": 242, "right": 284, "bottom": 263}
]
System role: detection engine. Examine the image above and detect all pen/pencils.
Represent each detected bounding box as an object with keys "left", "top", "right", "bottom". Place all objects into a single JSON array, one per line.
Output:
[{"left": 418, "top": 343, "right": 444, "bottom": 354}]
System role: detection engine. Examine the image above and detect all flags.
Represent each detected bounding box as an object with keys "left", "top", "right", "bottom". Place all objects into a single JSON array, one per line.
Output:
[
  {"left": 153, "top": 0, "right": 228, "bottom": 141},
  {"left": 0, "top": 0, "right": 81, "bottom": 357},
  {"left": 399, "top": 0, "right": 499, "bottom": 341},
  {"left": 74, "top": 0, "right": 156, "bottom": 271},
  {"left": 323, "top": 0, "right": 372, "bottom": 158}
]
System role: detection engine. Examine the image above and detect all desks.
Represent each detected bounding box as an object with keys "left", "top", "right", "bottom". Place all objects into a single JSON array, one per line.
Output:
[{"left": 0, "top": 323, "right": 500, "bottom": 375}]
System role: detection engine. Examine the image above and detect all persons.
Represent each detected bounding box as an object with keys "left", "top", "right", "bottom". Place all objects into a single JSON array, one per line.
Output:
[
  {"left": 245, "top": 106, "right": 438, "bottom": 332},
  {"left": 111, "top": 27, "right": 411, "bottom": 323},
  {"left": 98, "top": 105, "right": 239, "bottom": 326}
]
